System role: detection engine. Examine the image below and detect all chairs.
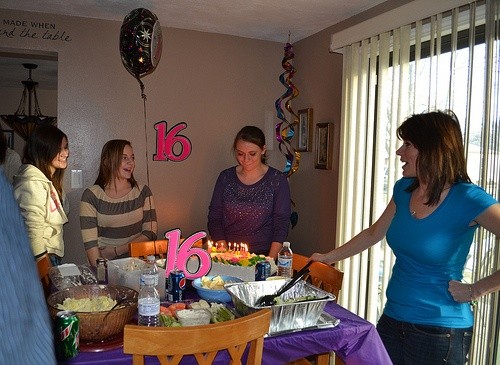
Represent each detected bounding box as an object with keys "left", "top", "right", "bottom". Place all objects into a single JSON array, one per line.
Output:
[
  {"left": 36, "top": 256, "right": 52, "bottom": 292},
  {"left": 128, "top": 239, "right": 202, "bottom": 259},
  {"left": 123, "top": 308, "right": 271, "bottom": 365},
  {"left": 285, "top": 253, "right": 344, "bottom": 365}
]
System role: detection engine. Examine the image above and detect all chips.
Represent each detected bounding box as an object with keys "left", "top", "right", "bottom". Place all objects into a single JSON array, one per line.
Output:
[{"left": 201, "top": 275, "right": 235, "bottom": 290}]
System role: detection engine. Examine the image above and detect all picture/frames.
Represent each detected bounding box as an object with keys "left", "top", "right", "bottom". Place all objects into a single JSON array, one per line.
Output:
[
  {"left": 296, "top": 108, "right": 313, "bottom": 153},
  {"left": 314, "top": 123, "right": 333, "bottom": 170},
  {"left": 1, "top": 130, "right": 14, "bottom": 150}
]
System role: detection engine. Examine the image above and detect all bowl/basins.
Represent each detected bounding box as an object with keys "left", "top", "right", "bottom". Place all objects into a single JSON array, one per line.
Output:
[
  {"left": 191, "top": 275, "right": 246, "bottom": 303},
  {"left": 47, "top": 283, "right": 139, "bottom": 344},
  {"left": 174, "top": 308, "right": 213, "bottom": 327}
]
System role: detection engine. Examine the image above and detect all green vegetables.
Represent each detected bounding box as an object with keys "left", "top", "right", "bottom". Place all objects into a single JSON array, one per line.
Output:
[{"left": 212, "top": 255, "right": 266, "bottom": 267}]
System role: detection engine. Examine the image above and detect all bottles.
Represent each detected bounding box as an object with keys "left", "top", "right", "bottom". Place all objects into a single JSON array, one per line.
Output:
[
  {"left": 95, "top": 258, "right": 107, "bottom": 285},
  {"left": 139, "top": 276, "right": 160, "bottom": 328},
  {"left": 140, "top": 256, "right": 158, "bottom": 290},
  {"left": 278, "top": 241, "right": 295, "bottom": 279}
]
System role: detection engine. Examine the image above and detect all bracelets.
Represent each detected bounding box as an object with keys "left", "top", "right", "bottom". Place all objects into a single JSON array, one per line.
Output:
[{"left": 114, "top": 247, "right": 119, "bottom": 257}]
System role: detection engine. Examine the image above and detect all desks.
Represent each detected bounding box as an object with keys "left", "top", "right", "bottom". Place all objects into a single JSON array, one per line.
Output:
[{"left": 60, "top": 265, "right": 393, "bottom": 365}]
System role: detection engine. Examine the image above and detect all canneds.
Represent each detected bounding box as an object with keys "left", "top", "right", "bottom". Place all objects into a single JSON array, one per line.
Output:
[
  {"left": 254, "top": 261, "right": 272, "bottom": 282},
  {"left": 56, "top": 309, "right": 80, "bottom": 359},
  {"left": 167, "top": 270, "right": 186, "bottom": 302}
]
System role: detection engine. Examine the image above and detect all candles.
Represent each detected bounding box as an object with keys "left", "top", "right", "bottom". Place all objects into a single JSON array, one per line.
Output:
[{"left": 207, "top": 240, "right": 248, "bottom": 257}]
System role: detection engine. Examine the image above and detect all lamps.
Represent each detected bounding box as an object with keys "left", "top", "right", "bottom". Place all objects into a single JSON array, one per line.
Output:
[{"left": 0, "top": 63, "right": 57, "bottom": 141}]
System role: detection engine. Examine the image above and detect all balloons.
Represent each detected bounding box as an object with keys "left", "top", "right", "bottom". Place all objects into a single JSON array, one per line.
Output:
[{"left": 119, "top": 8, "right": 163, "bottom": 80}]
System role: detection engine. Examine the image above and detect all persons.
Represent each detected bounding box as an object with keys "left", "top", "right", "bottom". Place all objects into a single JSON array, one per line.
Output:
[
  {"left": 307, "top": 112, "right": 500, "bottom": 365},
  {"left": 207, "top": 126, "right": 291, "bottom": 260},
  {"left": 79, "top": 139, "right": 158, "bottom": 268},
  {"left": 12, "top": 125, "right": 70, "bottom": 299}
]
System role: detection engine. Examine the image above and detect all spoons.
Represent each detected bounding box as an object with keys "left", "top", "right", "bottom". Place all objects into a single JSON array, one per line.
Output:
[{"left": 260, "top": 267, "right": 310, "bottom": 306}]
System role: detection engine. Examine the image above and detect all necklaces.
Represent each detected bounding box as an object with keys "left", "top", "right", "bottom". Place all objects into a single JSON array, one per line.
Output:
[{"left": 411, "top": 210, "right": 416, "bottom": 216}]
